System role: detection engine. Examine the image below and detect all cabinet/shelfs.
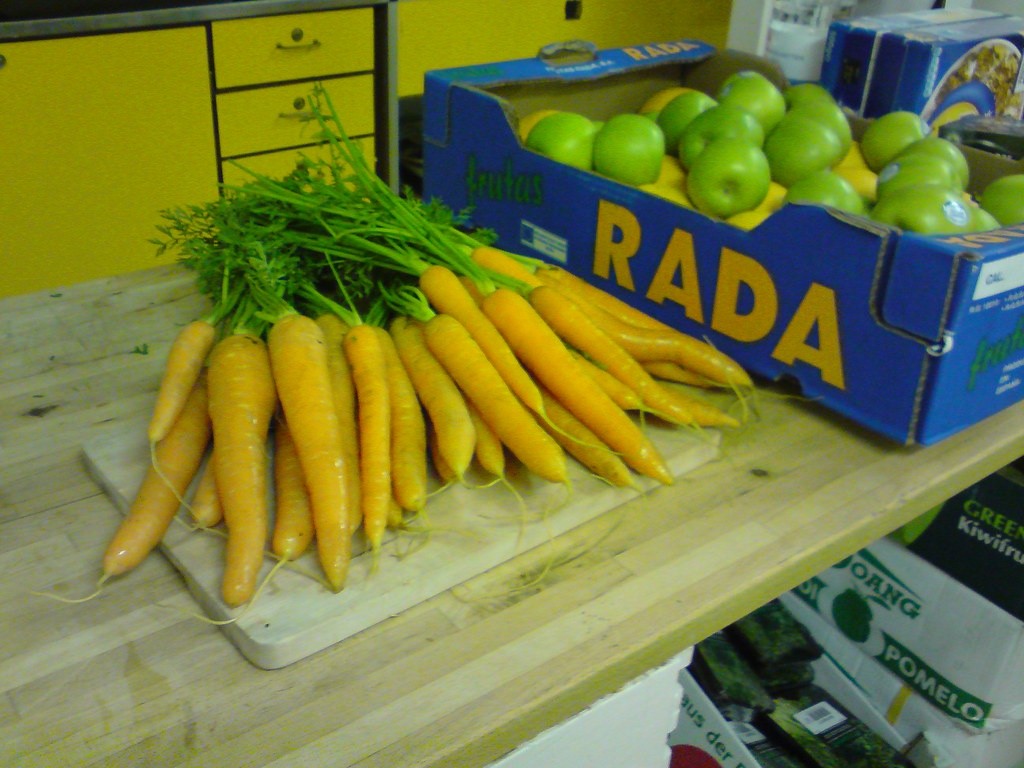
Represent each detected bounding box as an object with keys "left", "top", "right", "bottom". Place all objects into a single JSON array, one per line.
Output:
[{"left": 0, "top": 7, "right": 399, "bottom": 299}]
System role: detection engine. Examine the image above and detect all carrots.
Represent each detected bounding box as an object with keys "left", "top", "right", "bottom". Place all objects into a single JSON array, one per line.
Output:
[{"left": 94, "top": 249, "right": 755, "bottom": 622}]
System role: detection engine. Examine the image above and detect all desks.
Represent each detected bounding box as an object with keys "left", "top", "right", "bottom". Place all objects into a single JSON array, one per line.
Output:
[{"left": 0, "top": 259, "right": 1024, "bottom": 768}]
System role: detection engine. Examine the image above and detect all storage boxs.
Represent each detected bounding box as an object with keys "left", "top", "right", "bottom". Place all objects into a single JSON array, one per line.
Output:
[
  {"left": 883, "top": 467, "right": 1024, "bottom": 622},
  {"left": 791, "top": 536, "right": 1024, "bottom": 735},
  {"left": 738, "top": 723, "right": 803, "bottom": 768},
  {"left": 820, "top": 7, "right": 1004, "bottom": 118},
  {"left": 721, "top": 601, "right": 822, "bottom": 663},
  {"left": 752, "top": 682, "right": 915, "bottom": 768},
  {"left": 419, "top": 33, "right": 1024, "bottom": 452},
  {"left": 936, "top": 114, "right": 1024, "bottom": 160},
  {"left": 867, "top": 16, "right": 1024, "bottom": 131},
  {"left": 777, "top": 593, "right": 1024, "bottom": 768},
  {"left": 688, "top": 634, "right": 776, "bottom": 718},
  {"left": 667, "top": 670, "right": 759, "bottom": 768}
]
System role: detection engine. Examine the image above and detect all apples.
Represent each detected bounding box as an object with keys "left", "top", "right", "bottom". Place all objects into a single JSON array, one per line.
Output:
[{"left": 519, "top": 73, "right": 1024, "bottom": 235}]
